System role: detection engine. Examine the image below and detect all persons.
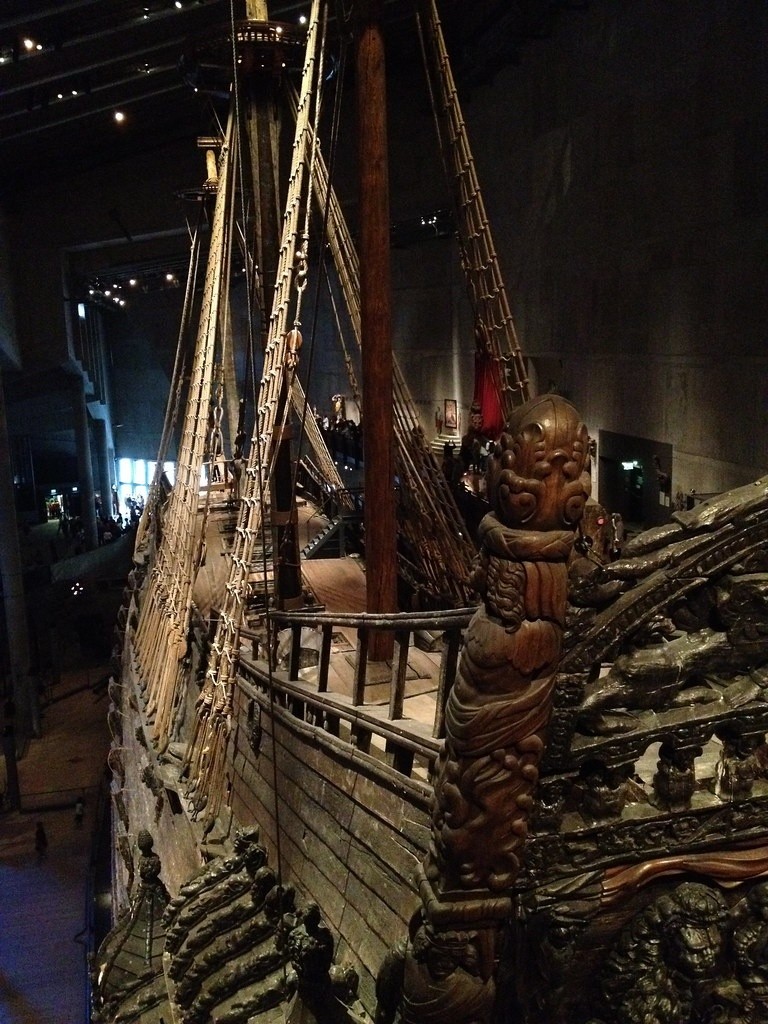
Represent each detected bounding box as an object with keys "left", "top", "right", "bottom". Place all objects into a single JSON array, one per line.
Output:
[
  {"left": 47, "top": 493, "right": 146, "bottom": 546},
  {"left": 442, "top": 440, "right": 457, "bottom": 460}
]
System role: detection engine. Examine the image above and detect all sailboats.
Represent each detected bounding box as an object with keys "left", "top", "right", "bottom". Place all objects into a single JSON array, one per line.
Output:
[{"left": 78, "top": 0, "right": 768, "bottom": 1024}]
